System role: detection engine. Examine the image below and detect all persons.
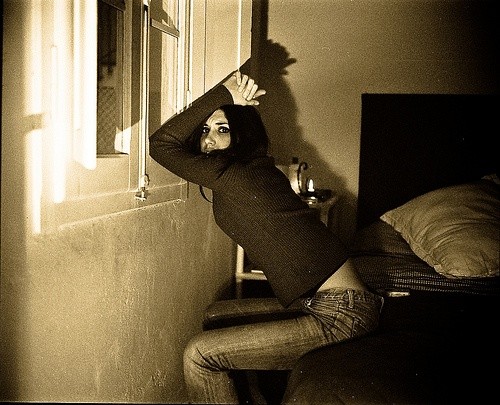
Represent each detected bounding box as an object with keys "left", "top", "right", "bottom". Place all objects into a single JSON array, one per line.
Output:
[{"left": 148, "top": 69, "right": 383, "bottom": 405}]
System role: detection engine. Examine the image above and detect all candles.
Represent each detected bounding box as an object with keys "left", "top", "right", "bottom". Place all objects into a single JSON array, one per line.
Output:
[{"left": 307, "top": 179, "right": 315, "bottom": 192}]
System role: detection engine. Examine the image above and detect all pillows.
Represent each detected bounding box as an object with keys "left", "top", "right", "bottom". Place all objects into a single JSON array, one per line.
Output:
[{"left": 379, "top": 172, "right": 500, "bottom": 280}]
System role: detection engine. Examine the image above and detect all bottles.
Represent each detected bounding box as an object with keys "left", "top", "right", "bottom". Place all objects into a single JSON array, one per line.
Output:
[{"left": 289, "top": 157, "right": 302, "bottom": 193}]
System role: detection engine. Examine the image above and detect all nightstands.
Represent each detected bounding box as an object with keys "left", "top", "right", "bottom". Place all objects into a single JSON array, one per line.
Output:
[{"left": 231, "top": 189, "right": 341, "bottom": 301}]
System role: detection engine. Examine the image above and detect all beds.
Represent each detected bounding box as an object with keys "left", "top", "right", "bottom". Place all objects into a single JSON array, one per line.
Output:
[{"left": 279, "top": 220, "right": 499, "bottom": 404}]
